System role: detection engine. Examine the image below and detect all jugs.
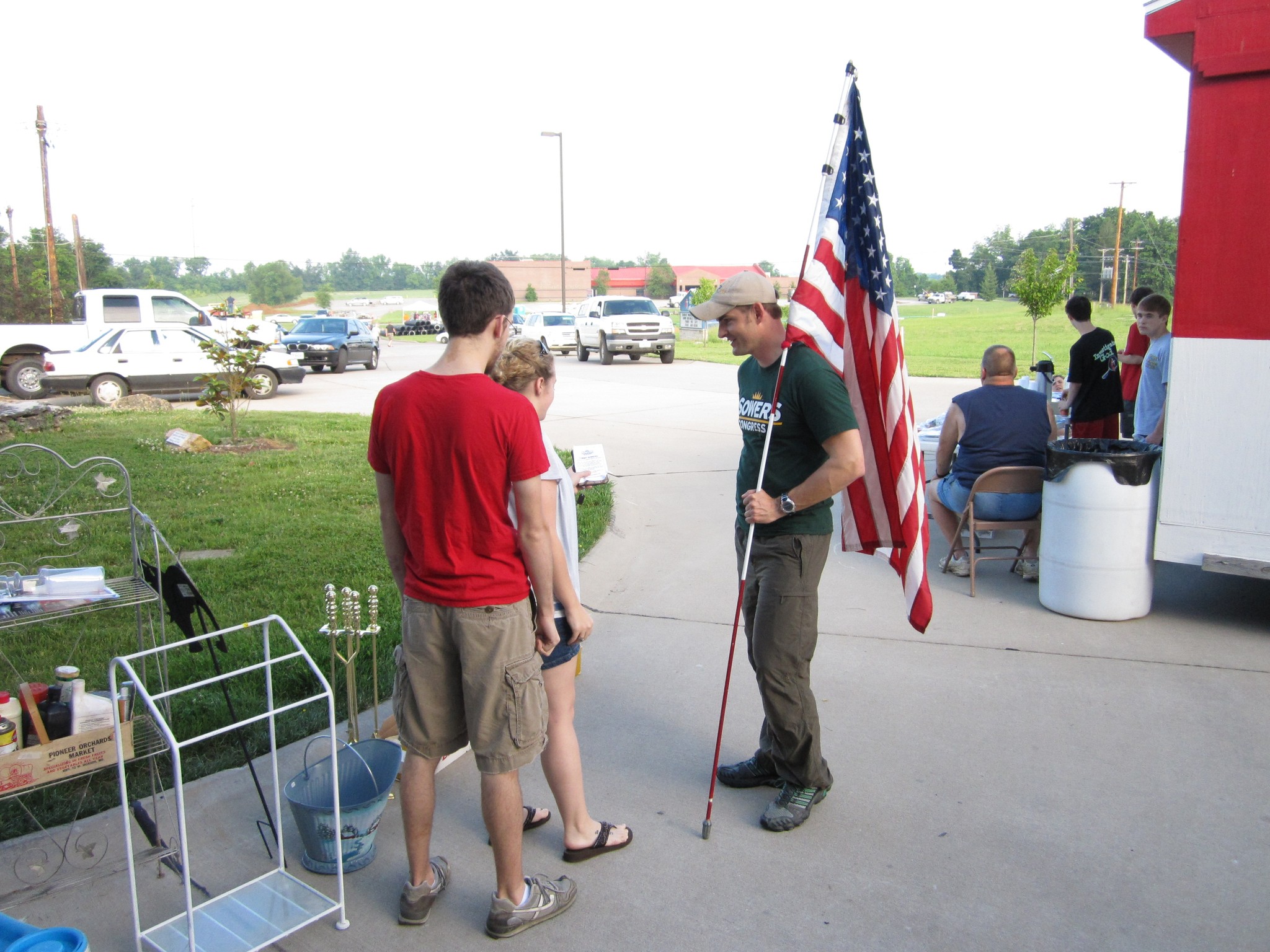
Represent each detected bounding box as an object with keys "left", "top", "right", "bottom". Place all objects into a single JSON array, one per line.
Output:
[{"left": 1029, "top": 360, "right": 1055, "bottom": 402}]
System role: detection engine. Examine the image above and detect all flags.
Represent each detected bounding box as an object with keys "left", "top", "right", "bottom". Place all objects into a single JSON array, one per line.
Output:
[{"left": 785, "top": 74, "right": 934, "bottom": 634}]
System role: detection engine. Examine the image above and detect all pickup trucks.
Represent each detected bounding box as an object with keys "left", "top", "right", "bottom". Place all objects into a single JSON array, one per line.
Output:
[
  {"left": 957, "top": 292, "right": 975, "bottom": 302},
  {"left": 0, "top": 289, "right": 287, "bottom": 399},
  {"left": 1008, "top": 293, "right": 1016, "bottom": 298},
  {"left": 918, "top": 291, "right": 957, "bottom": 304},
  {"left": 315, "top": 309, "right": 329, "bottom": 317}
]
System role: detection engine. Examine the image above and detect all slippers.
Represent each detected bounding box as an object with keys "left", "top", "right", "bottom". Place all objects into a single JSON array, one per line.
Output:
[
  {"left": 487, "top": 806, "right": 551, "bottom": 846},
  {"left": 561, "top": 817, "right": 631, "bottom": 862}
]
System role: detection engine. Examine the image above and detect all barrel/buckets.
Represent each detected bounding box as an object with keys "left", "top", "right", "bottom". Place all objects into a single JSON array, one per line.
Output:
[{"left": 283, "top": 735, "right": 403, "bottom": 875}]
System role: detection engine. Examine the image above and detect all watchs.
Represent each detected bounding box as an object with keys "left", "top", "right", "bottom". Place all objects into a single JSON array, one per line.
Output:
[{"left": 779, "top": 490, "right": 796, "bottom": 516}]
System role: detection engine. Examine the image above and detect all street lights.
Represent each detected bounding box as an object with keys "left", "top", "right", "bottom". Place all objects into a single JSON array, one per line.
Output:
[
  {"left": 914, "top": 285, "right": 916, "bottom": 296},
  {"left": 541, "top": 131, "right": 565, "bottom": 313}
]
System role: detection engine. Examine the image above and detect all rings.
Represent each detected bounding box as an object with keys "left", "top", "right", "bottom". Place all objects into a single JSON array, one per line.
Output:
[{"left": 578, "top": 636, "right": 585, "bottom": 643}]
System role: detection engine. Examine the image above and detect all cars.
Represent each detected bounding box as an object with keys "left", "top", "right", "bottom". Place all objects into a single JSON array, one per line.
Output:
[
  {"left": 265, "top": 314, "right": 300, "bottom": 323},
  {"left": 436, "top": 332, "right": 449, "bottom": 344},
  {"left": 282, "top": 317, "right": 380, "bottom": 374},
  {"left": 39, "top": 328, "right": 306, "bottom": 407},
  {"left": 509, "top": 311, "right": 577, "bottom": 354},
  {"left": 298, "top": 314, "right": 313, "bottom": 323}
]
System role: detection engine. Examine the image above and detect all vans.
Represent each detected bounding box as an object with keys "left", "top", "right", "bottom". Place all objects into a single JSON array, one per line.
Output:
[{"left": 574, "top": 295, "right": 676, "bottom": 365}]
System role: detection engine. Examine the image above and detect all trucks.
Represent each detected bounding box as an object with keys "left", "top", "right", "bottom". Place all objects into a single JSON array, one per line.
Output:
[{"left": 667, "top": 292, "right": 688, "bottom": 308}]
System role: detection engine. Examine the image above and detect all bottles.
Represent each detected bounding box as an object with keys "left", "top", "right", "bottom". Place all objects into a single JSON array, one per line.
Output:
[
  {"left": 0, "top": 691, "right": 24, "bottom": 750},
  {"left": 0, "top": 716, "right": 19, "bottom": 756},
  {"left": 117, "top": 687, "right": 131, "bottom": 723},
  {"left": 54, "top": 665, "right": 80, "bottom": 718},
  {"left": 1063, "top": 368, "right": 1070, "bottom": 389},
  {"left": 19, "top": 682, "right": 49, "bottom": 748},
  {"left": 37, "top": 684, "right": 71, "bottom": 741}
]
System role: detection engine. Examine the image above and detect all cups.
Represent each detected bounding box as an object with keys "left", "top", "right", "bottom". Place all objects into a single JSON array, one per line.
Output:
[
  {"left": 1021, "top": 376, "right": 1029, "bottom": 387},
  {"left": 1029, "top": 380, "right": 1035, "bottom": 391},
  {"left": 1018, "top": 379, "right": 1029, "bottom": 390}
]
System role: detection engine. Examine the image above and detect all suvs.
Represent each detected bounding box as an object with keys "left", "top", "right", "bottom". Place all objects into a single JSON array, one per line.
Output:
[
  {"left": 346, "top": 297, "right": 372, "bottom": 306},
  {"left": 379, "top": 296, "right": 404, "bottom": 305}
]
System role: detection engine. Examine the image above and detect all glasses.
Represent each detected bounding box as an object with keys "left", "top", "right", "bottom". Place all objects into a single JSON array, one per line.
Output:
[
  {"left": 536, "top": 339, "right": 548, "bottom": 358},
  {"left": 495, "top": 316, "right": 518, "bottom": 337}
]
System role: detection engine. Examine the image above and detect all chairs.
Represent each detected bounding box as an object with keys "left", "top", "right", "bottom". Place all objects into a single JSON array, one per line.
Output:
[{"left": 941, "top": 465, "right": 1052, "bottom": 597}]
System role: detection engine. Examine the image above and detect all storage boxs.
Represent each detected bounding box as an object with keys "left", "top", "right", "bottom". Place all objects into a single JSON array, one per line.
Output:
[{"left": 0, "top": 721, "right": 135, "bottom": 794}]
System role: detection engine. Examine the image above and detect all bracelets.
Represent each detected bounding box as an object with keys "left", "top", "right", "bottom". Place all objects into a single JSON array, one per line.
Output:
[{"left": 936, "top": 467, "right": 950, "bottom": 477}]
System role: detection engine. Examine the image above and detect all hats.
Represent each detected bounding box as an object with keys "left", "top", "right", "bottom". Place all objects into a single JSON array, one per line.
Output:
[{"left": 690, "top": 270, "right": 778, "bottom": 322}]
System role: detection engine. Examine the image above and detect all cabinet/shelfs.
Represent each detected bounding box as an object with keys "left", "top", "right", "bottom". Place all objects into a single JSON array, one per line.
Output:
[{"left": 0, "top": 444, "right": 187, "bottom": 914}]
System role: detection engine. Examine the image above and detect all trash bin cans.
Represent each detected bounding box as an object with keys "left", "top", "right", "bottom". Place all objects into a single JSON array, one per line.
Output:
[{"left": 1039, "top": 439, "right": 1163, "bottom": 620}]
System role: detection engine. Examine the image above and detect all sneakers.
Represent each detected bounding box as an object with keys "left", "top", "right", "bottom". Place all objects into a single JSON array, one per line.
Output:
[
  {"left": 1015, "top": 559, "right": 1040, "bottom": 579},
  {"left": 400, "top": 853, "right": 450, "bottom": 926},
  {"left": 938, "top": 553, "right": 973, "bottom": 577},
  {"left": 760, "top": 774, "right": 833, "bottom": 833},
  {"left": 716, "top": 756, "right": 784, "bottom": 791},
  {"left": 485, "top": 868, "right": 578, "bottom": 937}
]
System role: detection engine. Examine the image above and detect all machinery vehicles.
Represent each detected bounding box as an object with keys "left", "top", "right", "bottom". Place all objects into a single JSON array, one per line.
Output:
[{"left": 787, "top": 285, "right": 796, "bottom": 302}]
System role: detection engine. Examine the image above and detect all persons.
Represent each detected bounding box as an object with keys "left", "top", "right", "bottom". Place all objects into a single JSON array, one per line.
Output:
[
  {"left": 1052, "top": 376, "right": 1064, "bottom": 393},
  {"left": 1132, "top": 294, "right": 1172, "bottom": 446},
  {"left": 1058, "top": 296, "right": 1125, "bottom": 439},
  {"left": 688, "top": 268, "right": 867, "bottom": 831},
  {"left": 1117, "top": 287, "right": 1154, "bottom": 439},
  {"left": 368, "top": 260, "right": 632, "bottom": 941},
  {"left": 927, "top": 346, "right": 1059, "bottom": 580}
]
